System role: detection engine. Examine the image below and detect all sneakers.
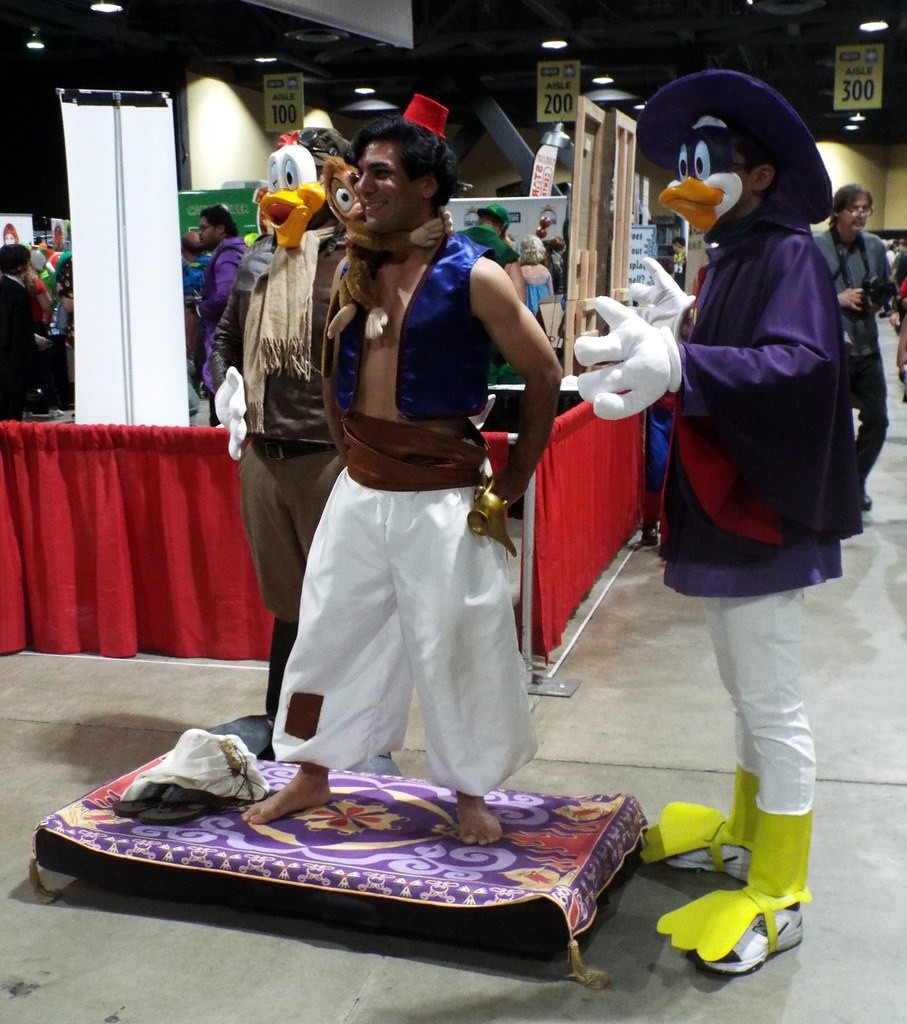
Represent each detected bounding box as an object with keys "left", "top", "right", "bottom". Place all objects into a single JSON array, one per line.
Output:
[
  {"left": 697, "top": 905, "right": 802, "bottom": 975},
  {"left": 666, "top": 844, "right": 752, "bottom": 881}
]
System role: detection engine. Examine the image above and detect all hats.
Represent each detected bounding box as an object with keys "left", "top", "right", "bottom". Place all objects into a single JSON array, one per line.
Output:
[
  {"left": 478, "top": 204, "right": 510, "bottom": 228},
  {"left": 637, "top": 71, "right": 832, "bottom": 224}
]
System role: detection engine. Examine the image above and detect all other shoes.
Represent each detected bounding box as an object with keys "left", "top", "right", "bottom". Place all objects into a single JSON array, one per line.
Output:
[{"left": 861, "top": 493, "right": 873, "bottom": 511}]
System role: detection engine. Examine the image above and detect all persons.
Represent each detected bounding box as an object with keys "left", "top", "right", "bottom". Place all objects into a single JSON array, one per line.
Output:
[
  {"left": 181, "top": 203, "right": 249, "bottom": 428},
  {"left": 575, "top": 69, "right": 863, "bottom": 976},
  {"left": 457, "top": 205, "right": 568, "bottom": 385},
  {"left": 812, "top": 182, "right": 892, "bottom": 511},
  {"left": 210, "top": 127, "right": 359, "bottom": 761},
  {"left": 642, "top": 389, "right": 676, "bottom": 546},
  {"left": 0, "top": 237, "right": 75, "bottom": 423},
  {"left": 240, "top": 94, "right": 564, "bottom": 845},
  {"left": 879, "top": 238, "right": 907, "bottom": 404},
  {"left": 673, "top": 236, "right": 685, "bottom": 292}
]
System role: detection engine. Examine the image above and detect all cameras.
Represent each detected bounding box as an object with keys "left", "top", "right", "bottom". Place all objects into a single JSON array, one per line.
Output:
[{"left": 855, "top": 273, "right": 899, "bottom": 318}]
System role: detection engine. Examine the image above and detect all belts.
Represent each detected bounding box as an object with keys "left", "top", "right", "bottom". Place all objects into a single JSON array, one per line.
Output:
[{"left": 254, "top": 439, "right": 336, "bottom": 460}]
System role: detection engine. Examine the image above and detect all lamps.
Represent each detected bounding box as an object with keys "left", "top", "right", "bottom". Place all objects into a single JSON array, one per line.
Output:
[
  {"left": 25, "top": 26, "right": 46, "bottom": 51},
  {"left": 539, "top": 122, "right": 572, "bottom": 151}
]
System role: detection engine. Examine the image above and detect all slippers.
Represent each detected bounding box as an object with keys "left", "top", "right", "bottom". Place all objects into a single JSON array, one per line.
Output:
[
  {"left": 140, "top": 787, "right": 237, "bottom": 826},
  {"left": 113, "top": 783, "right": 168, "bottom": 815}
]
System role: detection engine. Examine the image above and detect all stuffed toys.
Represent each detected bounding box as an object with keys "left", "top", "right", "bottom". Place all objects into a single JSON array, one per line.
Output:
[{"left": 322, "top": 156, "right": 454, "bottom": 339}]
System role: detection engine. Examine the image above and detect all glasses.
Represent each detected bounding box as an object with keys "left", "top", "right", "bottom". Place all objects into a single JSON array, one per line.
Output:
[
  {"left": 846, "top": 208, "right": 873, "bottom": 221},
  {"left": 200, "top": 223, "right": 211, "bottom": 231}
]
